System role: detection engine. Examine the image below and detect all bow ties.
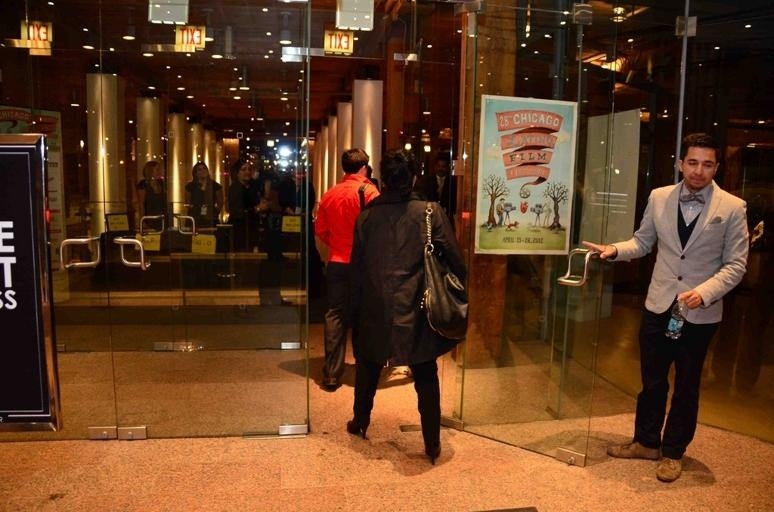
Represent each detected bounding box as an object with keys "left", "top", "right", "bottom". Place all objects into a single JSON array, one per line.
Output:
[{"left": 679, "top": 194, "right": 705, "bottom": 204}]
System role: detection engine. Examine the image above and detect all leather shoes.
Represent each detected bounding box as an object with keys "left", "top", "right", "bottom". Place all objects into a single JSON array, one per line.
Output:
[{"left": 323, "top": 375, "right": 337, "bottom": 390}]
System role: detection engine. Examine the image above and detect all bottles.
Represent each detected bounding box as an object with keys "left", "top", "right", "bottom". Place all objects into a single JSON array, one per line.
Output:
[{"left": 665, "top": 298, "right": 689, "bottom": 342}]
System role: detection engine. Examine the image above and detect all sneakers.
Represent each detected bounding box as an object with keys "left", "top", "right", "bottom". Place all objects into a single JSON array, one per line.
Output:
[{"left": 607, "top": 440, "right": 682, "bottom": 482}]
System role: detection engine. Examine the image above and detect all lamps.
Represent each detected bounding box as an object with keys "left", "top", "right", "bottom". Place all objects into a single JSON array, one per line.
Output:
[{"left": 613, "top": 7, "right": 626, "bottom": 23}]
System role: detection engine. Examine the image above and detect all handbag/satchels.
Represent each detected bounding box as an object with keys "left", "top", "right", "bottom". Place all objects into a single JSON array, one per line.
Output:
[{"left": 416, "top": 251, "right": 469, "bottom": 340}]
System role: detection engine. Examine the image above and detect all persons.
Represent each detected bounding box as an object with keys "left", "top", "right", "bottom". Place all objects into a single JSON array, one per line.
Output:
[
  {"left": 312, "top": 146, "right": 383, "bottom": 389},
  {"left": 701, "top": 143, "right": 774, "bottom": 402},
  {"left": 242, "top": 148, "right": 273, "bottom": 203},
  {"left": 423, "top": 150, "right": 457, "bottom": 214},
  {"left": 579, "top": 128, "right": 751, "bottom": 484},
  {"left": 226, "top": 158, "right": 271, "bottom": 252},
  {"left": 346, "top": 150, "right": 470, "bottom": 468},
  {"left": 278, "top": 164, "right": 316, "bottom": 251},
  {"left": 136, "top": 160, "right": 170, "bottom": 231},
  {"left": 184, "top": 161, "right": 224, "bottom": 228}
]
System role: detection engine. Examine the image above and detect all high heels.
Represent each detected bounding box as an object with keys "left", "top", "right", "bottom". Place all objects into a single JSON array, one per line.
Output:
[
  {"left": 347, "top": 419, "right": 370, "bottom": 440},
  {"left": 425, "top": 446, "right": 441, "bottom": 465}
]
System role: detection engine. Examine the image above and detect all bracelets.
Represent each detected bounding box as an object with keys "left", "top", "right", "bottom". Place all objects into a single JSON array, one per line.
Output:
[{"left": 256, "top": 205, "right": 261, "bottom": 212}]
